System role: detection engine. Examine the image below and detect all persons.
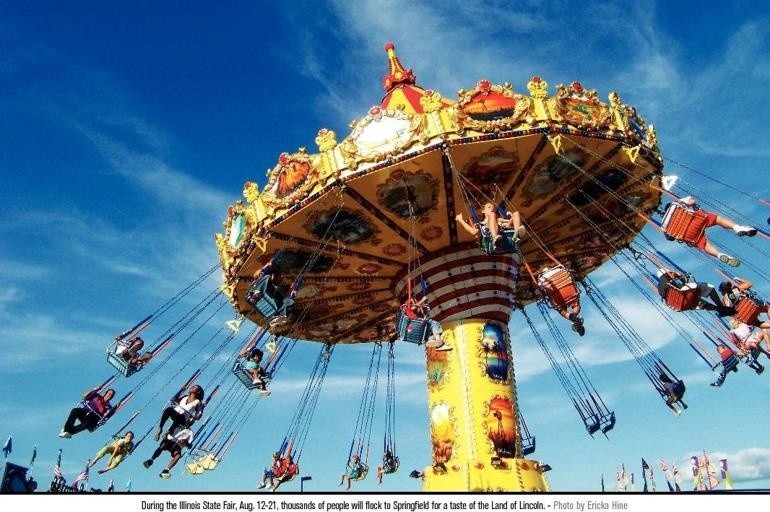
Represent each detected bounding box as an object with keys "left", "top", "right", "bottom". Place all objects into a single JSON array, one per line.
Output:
[
  {"left": 657, "top": 266, "right": 735, "bottom": 319},
  {"left": 156, "top": 383, "right": 206, "bottom": 447},
  {"left": 336, "top": 454, "right": 364, "bottom": 491},
  {"left": 709, "top": 345, "right": 740, "bottom": 388},
  {"left": 716, "top": 276, "right": 770, "bottom": 329},
  {"left": 537, "top": 264, "right": 585, "bottom": 335},
  {"left": 236, "top": 347, "right": 273, "bottom": 396},
  {"left": 454, "top": 201, "right": 527, "bottom": 255},
  {"left": 142, "top": 424, "right": 194, "bottom": 480},
  {"left": 114, "top": 329, "right": 147, "bottom": 369},
  {"left": 88, "top": 431, "right": 136, "bottom": 473},
  {"left": 661, "top": 195, "right": 759, "bottom": 267},
  {"left": 402, "top": 296, "right": 454, "bottom": 351},
  {"left": 660, "top": 372, "right": 687, "bottom": 415},
  {"left": 727, "top": 318, "right": 770, "bottom": 354},
  {"left": 270, "top": 454, "right": 299, "bottom": 492},
  {"left": 256, "top": 450, "right": 281, "bottom": 488},
  {"left": 58, "top": 385, "right": 117, "bottom": 438},
  {"left": 376, "top": 451, "right": 398, "bottom": 485},
  {"left": 249, "top": 257, "right": 298, "bottom": 331}
]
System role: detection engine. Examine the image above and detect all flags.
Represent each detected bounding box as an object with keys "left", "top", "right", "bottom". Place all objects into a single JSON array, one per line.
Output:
[
  {"left": 2, "top": 434, "right": 132, "bottom": 493},
  {"left": 599, "top": 448, "right": 735, "bottom": 492}
]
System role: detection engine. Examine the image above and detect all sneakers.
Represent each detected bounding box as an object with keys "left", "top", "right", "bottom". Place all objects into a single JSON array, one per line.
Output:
[
  {"left": 88, "top": 460, "right": 97, "bottom": 468},
  {"left": 512, "top": 224, "right": 526, "bottom": 244},
  {"left": 153, "top": 428, "right": 163, "bottom": 440},
  {"left": 270, "top": 315, "right": 291, "bottom": 327},
  {"left": 159, "top": 469, "right": 171, "bottom": 479},
  {"left": 675, "top": 412, "right": 681, "bottom": 417},
  {"left": 426, "top": 339, "right": 454, "bottom": 352},
  {"left": 138, "top": 350, "right": 152, "bottom": 361},
  {"left": 98, "top": 469, "right": 108, "bottom": 474},
  {"left": 249, "top": 291, "right": 262, "bottom": 305},
  {"left": 58, "top": 429, "right": 71, "bottom": 439},
  {"left": 128, "top": 343, "right": 143, "bottom": 355},
  {"left": 718, "top": 306, "right": 738, "bottom": 317},
  {"left": 143, "top": 459, "right": 153, "bottom": 469},
  {"left": 710, "top": 382, "right": 719, "bottom": 386},
  {"left": 683, "top": 405, "right": 687, "bottom": 409},
  {"left": 735, "top": 226, "right": 758, "bottom": 237},
  {"left": 568, "top": 313, "right": 584, "bottom": 336},
  {"left": 756, "top": 366, "right": 764, "bottom": 376},
  {"left": 718, "top": 254, "right": 740, "bottom": 268},
  {"left": 257, "top": 481, "right": 275, "bottom": 492},
  {"left": 493, "top": 235, "right": 506, "bottom": 252},
  {"left": 259, "top": 389, "right": 272, "bottom": 397},
  {"left": 252, "top": 377, "right": 264, "bottom": 386}
]
